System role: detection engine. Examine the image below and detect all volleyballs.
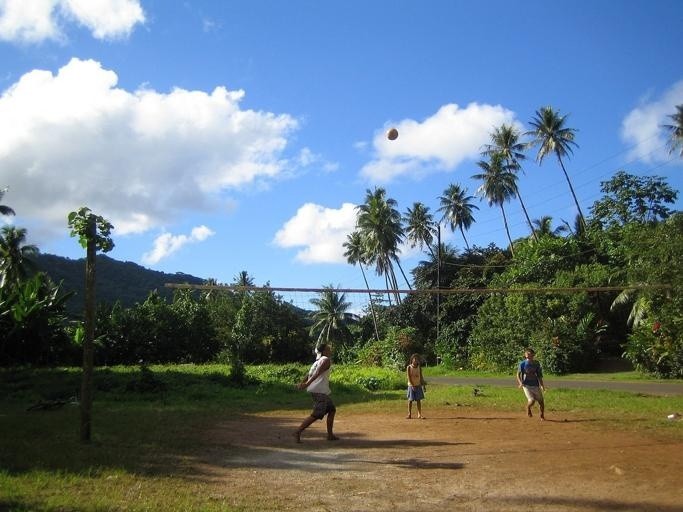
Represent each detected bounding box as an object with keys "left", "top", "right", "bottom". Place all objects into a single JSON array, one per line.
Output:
[{"left": 387, "top": 128, "right": 398, "bottom": 140}]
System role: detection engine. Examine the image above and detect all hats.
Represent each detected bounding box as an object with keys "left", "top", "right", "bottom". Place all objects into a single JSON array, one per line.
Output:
[{"left": 313, "top": 342, "right": 326, "bottom": 362}]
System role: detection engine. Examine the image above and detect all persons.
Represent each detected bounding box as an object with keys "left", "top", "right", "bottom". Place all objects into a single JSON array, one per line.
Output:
[
  {"left": 290, "top": 343, "right": 339, "bottom": 443},
  {"left": 516, "top": 349, "right": 547, "bottom": 421},
  {"left": 404, "top": 354, "right": 426, "bottom": 419}
]
